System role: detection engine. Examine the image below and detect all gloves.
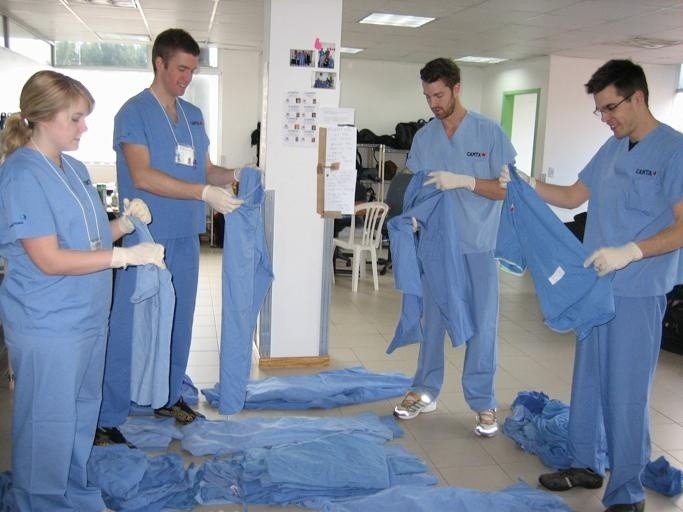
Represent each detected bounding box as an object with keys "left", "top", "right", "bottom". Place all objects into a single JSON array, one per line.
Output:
[
  {"left": 583, "top": 242, "right": 643, "bottom": 277},
  {"left": 235, "top": 166, "right": 260, "bottom": 183},
  {"left": 111, "top": 243, "right": 166, "bottom": 269},
  {"left": 423, "top": 171, "right": 476, "bottom": 191},
  {"left": 202, "top": 185, "right": 242, "bottom": 214},
  {"left": 499, "top": 166, "right": 535, "bottom": 192},
  {"left": 119, "top": 200, "right": 151, "bottom": 234}
]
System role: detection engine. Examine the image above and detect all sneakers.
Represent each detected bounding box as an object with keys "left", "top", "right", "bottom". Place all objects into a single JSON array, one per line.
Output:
[
  {"left": 394, "top": 393, "right": 436, "bottom": 419},
  {"left": 153, "top": 401, "right": 204, "bottom": 424},
  {"left": 604, "top": 501, "right": 645, "bottom": 512},
  {"left": 92, "top": 428, "right": 137, "bottom": 451},
  {"left": 474, "top": 406, "right": 498, "bottom": 437},
  {"left": 539, "top": 468, "right": 602, "bottom": 491}
]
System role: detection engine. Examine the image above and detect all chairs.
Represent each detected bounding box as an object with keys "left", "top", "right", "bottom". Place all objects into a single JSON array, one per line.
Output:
[{"left": 332, "top": 202, "right": 388, "bottom": 293}]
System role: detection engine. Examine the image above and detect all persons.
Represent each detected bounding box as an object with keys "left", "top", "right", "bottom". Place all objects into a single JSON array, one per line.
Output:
[
  {"left": 113, "top": 29, "right": 264, "bottom": 424},
  {"left": 499, "top": 59, "right": 683, "bottom": 512},
  {"left": 391, "top": 57, "right": 515, "bottom": 439},
  {"left": 0, "top": 70, "right": 165, "bottom": 512}
]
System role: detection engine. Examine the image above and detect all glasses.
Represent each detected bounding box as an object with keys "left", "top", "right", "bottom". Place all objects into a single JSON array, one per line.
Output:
[{"left": 592, "top": 91, "right": 636, "bottom": 119}]
